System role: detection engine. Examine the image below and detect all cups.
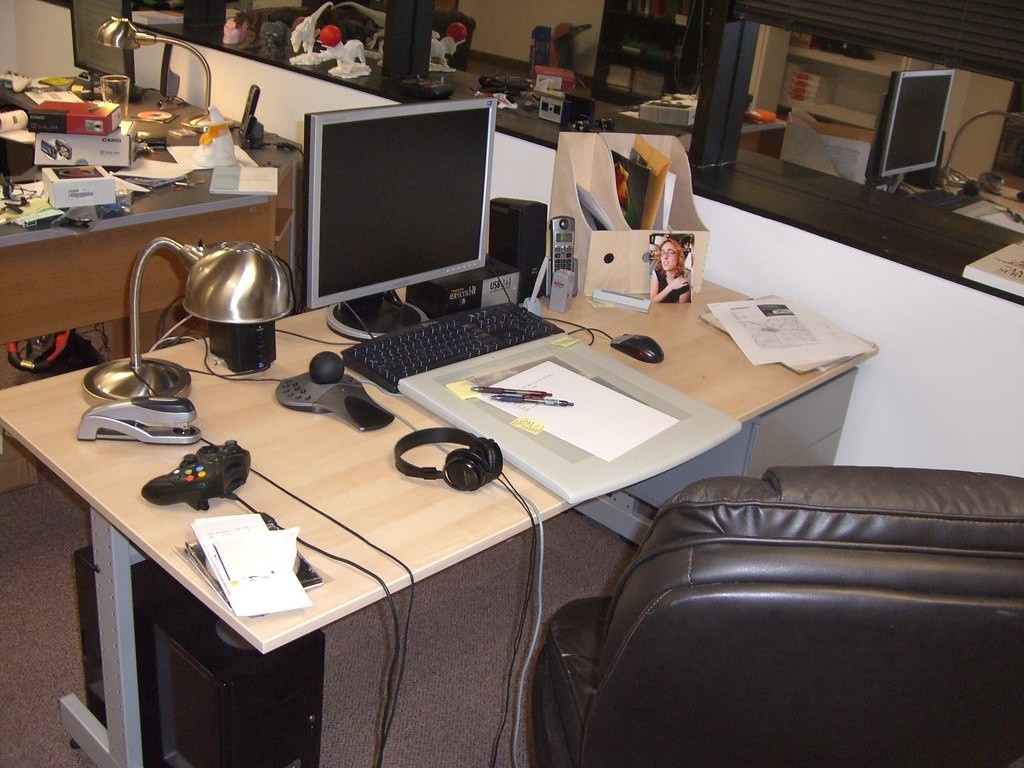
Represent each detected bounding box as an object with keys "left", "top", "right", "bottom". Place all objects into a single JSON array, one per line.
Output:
[{"left": 100, "top": 75, "right": 130, "bottom": 120}]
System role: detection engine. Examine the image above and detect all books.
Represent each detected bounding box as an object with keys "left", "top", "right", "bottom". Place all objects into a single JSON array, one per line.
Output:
[
  {"left": 626, "top": 0, "right": 691, "bottom": 18},
  {"left": 126, "top": 177, "right": 186, "bottom": 187},
  {"left": 963, "top": 240, "right": 1024, "bottom": 298},
  {"left": 171, "top": 523, "right": 323, "bottom": 609},
  {"left": 622, "top": 41, "right": 647, "bottom": 52},
  {"left": 210, "top": 165, "right": 278, "bottom": 195},
  {"left": 132, "top": 11, "right": 183, "bottom": 26}
]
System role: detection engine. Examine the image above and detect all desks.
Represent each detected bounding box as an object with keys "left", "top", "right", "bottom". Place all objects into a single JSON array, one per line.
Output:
[
  {"left": 0, "top": 73, "right": 303, "bottom": 361},
  {"left": 0, "top": 277, "right": 878, "bottom": 768},
  {"left": 619, "top": 109, "right": 787, "bottom": 161},
  {"left": 901, "top": 175, "right": 1024, "bottom": 221}
]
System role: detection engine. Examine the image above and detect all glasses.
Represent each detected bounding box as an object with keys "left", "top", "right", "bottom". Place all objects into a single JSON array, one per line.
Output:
[{"left": 660, "top": 249, "right": 679, "bottom": 258}]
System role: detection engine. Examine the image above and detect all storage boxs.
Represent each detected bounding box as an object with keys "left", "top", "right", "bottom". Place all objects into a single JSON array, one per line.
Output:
[
  {"left": 533, "top": 65, "right": 575, "bottom": 91},
  {"left": 1, "top": 345, "right": 38, "bottom": 493},
  {"left": 28, "top": 100, "right": 122, "bottom": 137},
  {"left": 639, "top": 99, "right": 696, "bottom": 127},
  {"left": 34, "top": 122, "right": 138, "bottom": 166},
  {"left": 42, "top": 167, "right": 117, "bottom": 210}
]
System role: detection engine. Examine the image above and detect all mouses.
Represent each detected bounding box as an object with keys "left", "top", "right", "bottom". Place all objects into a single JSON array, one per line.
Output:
[{"left": 964, "top": 179, "right": 979, "bottom": 196}]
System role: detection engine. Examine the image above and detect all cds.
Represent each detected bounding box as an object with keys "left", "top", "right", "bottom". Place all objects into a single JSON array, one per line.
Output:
[{"left": 138, "top": 110, "right": 173, "bottom": 120}]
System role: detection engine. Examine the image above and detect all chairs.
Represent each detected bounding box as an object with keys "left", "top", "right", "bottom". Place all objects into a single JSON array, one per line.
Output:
[{"left": 530, "top": 463, "right": 1023, "bottom": 768}]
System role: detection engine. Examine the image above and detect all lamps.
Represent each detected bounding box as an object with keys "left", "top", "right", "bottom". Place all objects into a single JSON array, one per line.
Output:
[
  {"left": 95, "top": 16, "right": 233, "bottom": 132},
  {"left": 81, "top": 238, "right": 296, "bottom": 408},
  {"left": 938, "top": 109, "right": 1024, "bottom": 187}
]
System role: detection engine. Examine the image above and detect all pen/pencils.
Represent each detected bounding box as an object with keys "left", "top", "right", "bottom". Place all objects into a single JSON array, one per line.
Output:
[
  {"left": 490, "top": 393, "right": 573, "bottom": 409},
  {"left": 470, "top": 383, "right": 551, "bottom": 396},
  {"left": 1007, "top": 207, "right": 1020, "bottom": 222},
  {"left": 174, "top": 179, "right": 198, "bottom": 191}
]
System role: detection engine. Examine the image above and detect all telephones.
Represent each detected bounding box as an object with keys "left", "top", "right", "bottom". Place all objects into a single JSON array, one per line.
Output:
[
  {"left": 544, "top": 214, "right": 581, "bottom": 301},
  {"left": 240, "top": 84, "right": 261, "bottom": 132}
]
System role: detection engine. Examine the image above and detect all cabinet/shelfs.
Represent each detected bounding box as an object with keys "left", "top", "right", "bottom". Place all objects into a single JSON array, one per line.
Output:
[
  {"left": 777, "top": 44, "right": 934, "bottom": 130},
  {"left": 590, "top": 0, "right": 713, "bottom": 108}
]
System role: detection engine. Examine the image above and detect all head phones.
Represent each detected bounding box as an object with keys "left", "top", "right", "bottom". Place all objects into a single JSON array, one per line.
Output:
[{"left": 393, "top": 427, "right": 504, "bottom": 491}]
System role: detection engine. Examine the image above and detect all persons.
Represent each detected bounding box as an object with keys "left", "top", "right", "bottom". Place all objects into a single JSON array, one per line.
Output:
[{"left": 651, "top": 239, "right": 691, "bottom": 303}]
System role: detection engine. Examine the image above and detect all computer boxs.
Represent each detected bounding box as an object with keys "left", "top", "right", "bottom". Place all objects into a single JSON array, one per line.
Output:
[{"left": 74, "top": 545, "right": 324, "bottom": 768}]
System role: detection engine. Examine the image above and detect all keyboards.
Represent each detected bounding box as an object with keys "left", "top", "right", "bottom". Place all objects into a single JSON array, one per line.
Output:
[
  {"left": 910, "top": 190, "right": 971, "bottom": 211},
  {"left": 341, "top": 301, "right": 565, "bottom": 393},
  {"left": 609, "top": 333, "right": 665, "bottom": 364}
]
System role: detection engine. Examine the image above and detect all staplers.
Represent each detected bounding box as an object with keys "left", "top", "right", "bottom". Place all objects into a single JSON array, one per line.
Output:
[{"left": 77, "top": 395, "right": 202, "bottom": 445}]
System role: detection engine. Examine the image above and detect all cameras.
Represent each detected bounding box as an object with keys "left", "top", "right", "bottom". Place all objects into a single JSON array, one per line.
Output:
[
  {"left": 41, "top": 138, "right": 72, "bottom": 160},
  {"left": 85, "top": 119, "right": 104, "bottom": 132}
]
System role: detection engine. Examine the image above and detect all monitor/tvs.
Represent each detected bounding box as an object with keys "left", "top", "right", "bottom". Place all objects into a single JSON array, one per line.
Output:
[
  {"left": 70, "top": 0, "right": 143, "bottom": 100},
  {"left": 301, "top": 96, "right": 497, "bottom": 340},
  {"left": 863, "top": 68, "right": 955, "bottom": 194}
]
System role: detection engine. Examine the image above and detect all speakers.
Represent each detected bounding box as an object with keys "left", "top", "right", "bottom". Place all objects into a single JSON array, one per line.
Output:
[
  {"left": 489, "top": 197, "right": 548, "bottom": 303},
  {"left": 208, "top": 321, "right": 277, "bottom": 373}
]
